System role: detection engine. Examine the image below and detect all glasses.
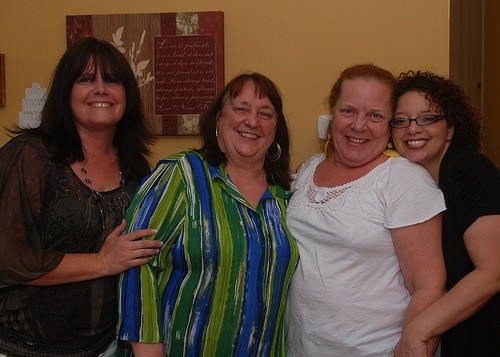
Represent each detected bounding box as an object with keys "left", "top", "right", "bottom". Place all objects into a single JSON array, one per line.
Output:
[{"left": 388, "top": 114, "right": 446, "bottom": 128}]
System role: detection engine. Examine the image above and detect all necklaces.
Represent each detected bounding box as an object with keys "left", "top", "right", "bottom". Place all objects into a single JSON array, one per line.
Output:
[{"left": 78, "top": 156, "right": 129, "bottom": 255}]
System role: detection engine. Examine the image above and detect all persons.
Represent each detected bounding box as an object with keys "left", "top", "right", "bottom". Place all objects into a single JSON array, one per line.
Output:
[
  {"left": 286, "top": 64, "right": 448, "bottom": 357},
  {"left": 0, "top": 35, "right": 152, "bottom": 357},
  {"left": 388, "top": 69, "right": 500, "bottom": 357},
  {"left": 113, "top": 72, "right": 300, "bottom": 357}
]
